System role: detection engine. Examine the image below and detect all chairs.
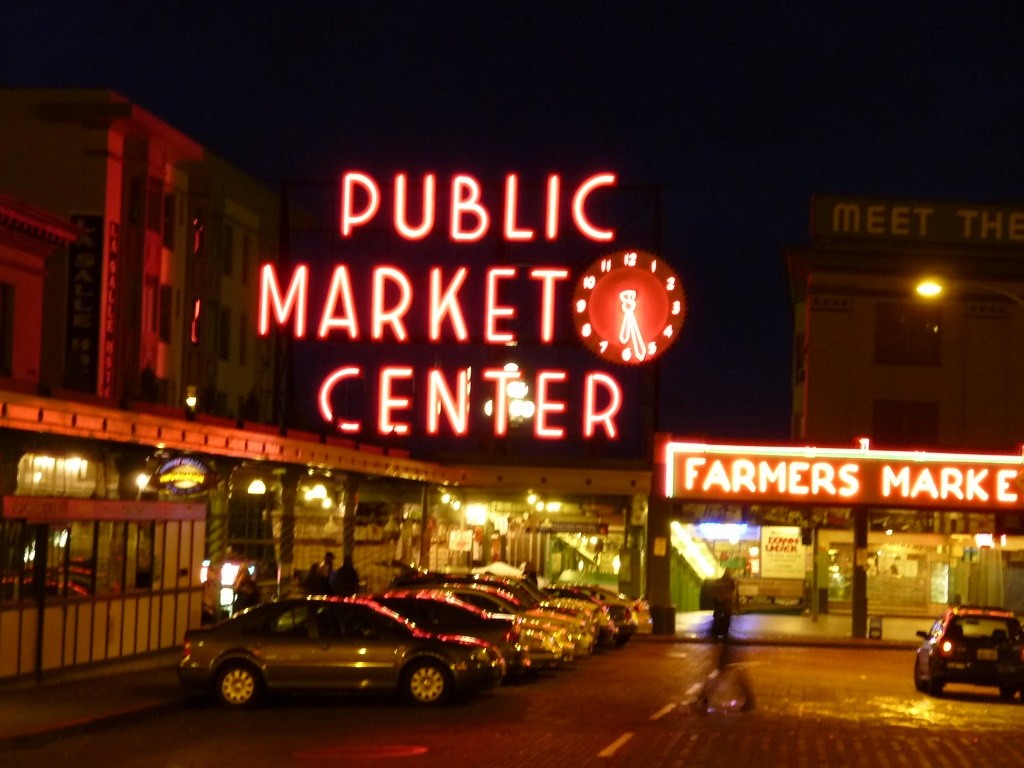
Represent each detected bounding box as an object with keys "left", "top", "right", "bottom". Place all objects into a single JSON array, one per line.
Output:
[
  {"left": 949, "top": 623, "right": 963, "bottom": 636},
  {"left": 992, "top": 629, "right": 1006, "bottom": 638}
]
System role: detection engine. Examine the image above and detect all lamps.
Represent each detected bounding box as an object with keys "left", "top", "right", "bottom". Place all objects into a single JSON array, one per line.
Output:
[{"left": 245, "top": 467, "right": 268, "bottom": 496}]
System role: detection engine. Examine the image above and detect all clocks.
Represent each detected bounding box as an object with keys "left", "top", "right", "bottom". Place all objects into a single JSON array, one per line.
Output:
[{"left": 573, "top": 249, "right": 689, "bottom": 364}]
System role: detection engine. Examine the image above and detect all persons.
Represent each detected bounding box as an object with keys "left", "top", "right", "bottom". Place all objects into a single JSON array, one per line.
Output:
[
  {"left": 279, "top": 552, "right": 358, "bottom": 597},
  {"left": 233, "top": 580, "right": 258, "bottom": 615},
  {"left": 525, "top": 571, "right": 537, "bottom": 590},
  {"left": 712, "top": 568, "right": 735, "bottom": 636}
]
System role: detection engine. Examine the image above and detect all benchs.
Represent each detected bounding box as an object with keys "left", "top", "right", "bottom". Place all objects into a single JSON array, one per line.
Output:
[{"left": 867, "top": 608, "right": 940, "bottom": 641}]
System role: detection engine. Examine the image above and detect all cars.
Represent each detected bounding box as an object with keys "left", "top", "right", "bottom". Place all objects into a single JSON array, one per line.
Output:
[
  {"left": 913, "top": 603, "right": 1024, "bottom": 703},
  {"left": 175, "top": 594, "right": 506, "bottom": 709},
  {"left": 279, "top": 566, "right": 653, "bottom": 686},
  {"left": 0, "top": 550, "right": 121, "bottom": 606}
]
